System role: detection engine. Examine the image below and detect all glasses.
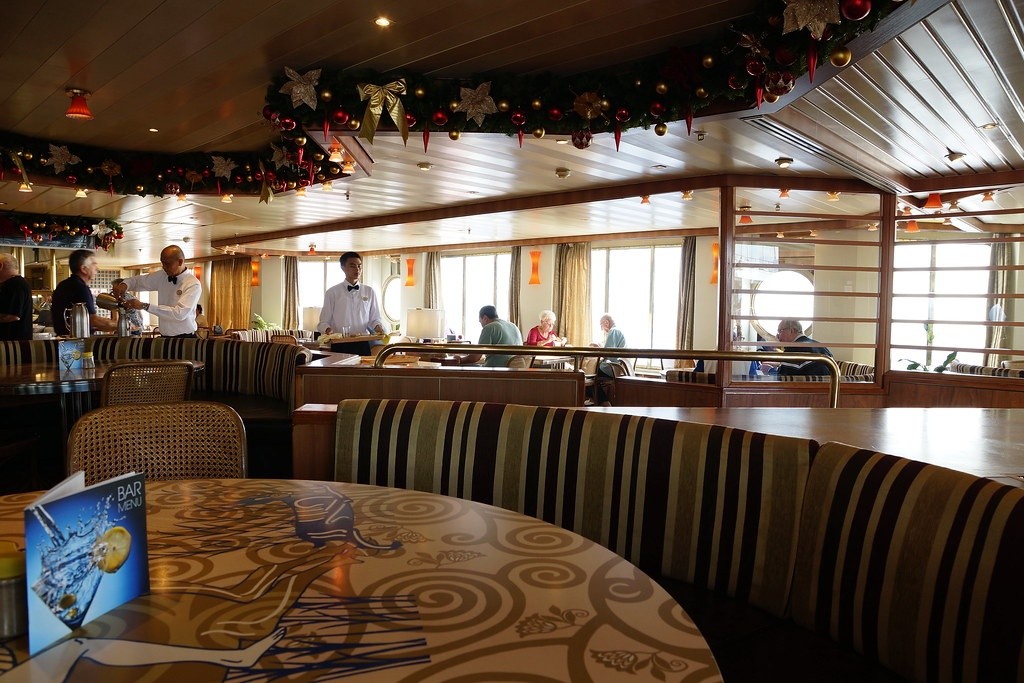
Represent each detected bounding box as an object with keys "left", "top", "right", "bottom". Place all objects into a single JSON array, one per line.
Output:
[{"left": 777, "top": 327, "right": 789, "bottom": 335}]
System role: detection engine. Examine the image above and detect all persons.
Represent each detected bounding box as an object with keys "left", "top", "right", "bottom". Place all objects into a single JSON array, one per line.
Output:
[
  {"left": 527, "top": 310, "right": 559, "bottom": 346},
  {"left": 0, "top": 253, "right": 33, "bottom": 342},
  {"left": 748, "top": 319, "right": 834, "bottom": 376},
  {"left": 111, "top": 245, "right": 202, "bottom": 338},
  {"left": 584, "top": 313, "right": 626, "bottom": 405},
  {"left": 195, "top": 304, "right": 208, "bottom": 338},
  {"left": 52, "top": 250, "right": 119, "bottom": 338},
  {"left": 454, "top": 305, "right": 524, "bottom": 367},
  {"left": 317, "top": 251, "right": 392, "bottom": 355}
]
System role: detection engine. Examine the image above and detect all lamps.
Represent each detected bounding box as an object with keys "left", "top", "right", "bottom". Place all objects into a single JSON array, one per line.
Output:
[
  {"left": 405, "top": 258, "right": 416, "bottom": 287},
  {"left": 251, "top": 261, "right": 259, "bottom": 286},
  {"left": 194, "top": 266, "right": 201, "bottom": 281},
  {"left": 308, "top": 245, "right": 317, "bottom": 255},
  {"left": 18, "top": 85, "right": 1001, "bottom": 240},
  {"left": 528, "top": 251, "right": 542, "bottom": 285},
  {"left": 710, "top": 242, "right": 718, "bottom": 286}
]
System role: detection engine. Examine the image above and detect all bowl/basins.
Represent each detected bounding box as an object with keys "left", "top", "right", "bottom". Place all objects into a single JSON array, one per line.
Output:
[
  {"left": 33, "top": 325, "right": 45, "bottom": 333},
  {"left": 32, "top": 314, "right": 39, "bottom": 322}
]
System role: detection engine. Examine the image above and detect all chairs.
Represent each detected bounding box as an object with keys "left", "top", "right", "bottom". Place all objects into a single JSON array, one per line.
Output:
[
  {"left": 66, "top": 400, "right": 247, "bottom": 487},
  {"left": 271, "top": 335, "right": 299, "bottom": 346},
  {"left": 194, "top": 329, "right": 210, "bottom": 338},
  {"left": 152, "top": 327, "right": 159, "bottom": 335},
  {"left": 225, "top": 329, "right": 247, "bottom": 335},
  {"left": 100, "top": 362, "right": 193, "bottom": 409},
  {"left": 507, "top": 355, "right": 526, "bottom": 368},
  {"left": 578, "top": 343, "right": 600, "bottom": 407}
]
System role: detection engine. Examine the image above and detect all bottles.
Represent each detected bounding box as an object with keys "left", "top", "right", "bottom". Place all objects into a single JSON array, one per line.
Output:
[{"left": 81, "top": 352, "right": 95, "bottom": 369}]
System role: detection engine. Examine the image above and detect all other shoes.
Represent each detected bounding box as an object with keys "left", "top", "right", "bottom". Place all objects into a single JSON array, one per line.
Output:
[{"left": 584, "top": 399, "right": 594, "bottom": 406}]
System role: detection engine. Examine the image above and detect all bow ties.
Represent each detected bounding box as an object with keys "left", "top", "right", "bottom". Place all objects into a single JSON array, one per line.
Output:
[
  {"left": 347, "top": 285, "right": 360, "bottom": 292},
  {"left": 168, "top": 276, "right": 178, "bottom": 283}
]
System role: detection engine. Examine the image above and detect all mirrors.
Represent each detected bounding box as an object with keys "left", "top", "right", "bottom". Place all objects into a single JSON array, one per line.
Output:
[{"left": 748, "top": 268, "right": 814, "bottom": 342}]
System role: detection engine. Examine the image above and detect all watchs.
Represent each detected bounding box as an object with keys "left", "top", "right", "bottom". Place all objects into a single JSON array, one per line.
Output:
[{"left": 143, "top": 303, "right": 148, "bottom": 310}]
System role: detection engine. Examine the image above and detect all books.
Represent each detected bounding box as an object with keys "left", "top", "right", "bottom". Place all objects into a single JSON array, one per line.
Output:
[
  {"left": 24, "top": 470, "right": 151, "bottom": 657},
  {"left": 59, "top": 340, "right": 84, "bottom": 369},
  {"left": 60, "top": 369, "right": 84, "bottom": 380}
]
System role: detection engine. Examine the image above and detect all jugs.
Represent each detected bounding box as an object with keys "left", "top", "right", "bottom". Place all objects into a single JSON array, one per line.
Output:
[
  {"left": 96, "top": 292, "right": 131, "bottom": 338},
  {"left": 64, "top": 302, "right": 90, "bottom": 339}
]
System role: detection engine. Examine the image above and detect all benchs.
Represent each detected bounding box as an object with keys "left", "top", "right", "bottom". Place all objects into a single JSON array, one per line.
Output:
[{"left": 0, "top": 328, "right": 1024, "bottom": 683}]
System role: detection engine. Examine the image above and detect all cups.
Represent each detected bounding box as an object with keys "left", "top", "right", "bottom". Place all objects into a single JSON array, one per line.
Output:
[{"left": 342, "top": 325, "right": 350, "bottom": 339}]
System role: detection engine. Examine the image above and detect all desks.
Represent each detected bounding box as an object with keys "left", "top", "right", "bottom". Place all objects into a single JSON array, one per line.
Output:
[
  {"left": 0, "top": 359, "right": 205, "bottom": 443},
  {"left": 529, "top": 355, "right": 576, "bottom": 369},
  {"left": 0, "top": 479, "right": 724, "bottom": 683}
]
